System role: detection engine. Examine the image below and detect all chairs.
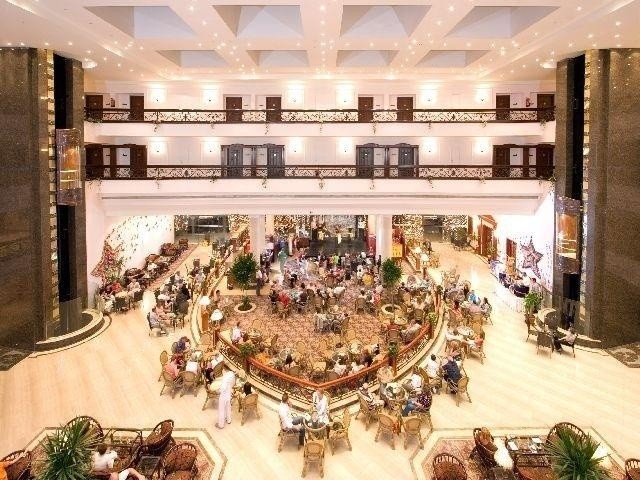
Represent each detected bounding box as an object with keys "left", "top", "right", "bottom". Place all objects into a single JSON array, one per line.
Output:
[
  {"left": 0, "top": 418, "right": 203, "bottom": 479},
  {"left": 494, "top": 267, "right": 579, "bottom": 357},
  {"left": 431, "top": 421, "right": 640, "bottom": 480}
]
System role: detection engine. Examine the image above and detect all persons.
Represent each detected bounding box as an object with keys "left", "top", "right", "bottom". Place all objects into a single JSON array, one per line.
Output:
[
  {"left": 107, "top": 469, "right": 145, "bottom": 480},
  {"left": 553, "top": 325, "right": 577, "bottom": 354},
  {"left": 528, "top": 276, "right": 541, "bottom": 313},
  {"left": 104, "top": 228, "right": 492, "bottom": 448},
  {"left": 91, "top": 445, "right": 119, "bottom": 471}
]
